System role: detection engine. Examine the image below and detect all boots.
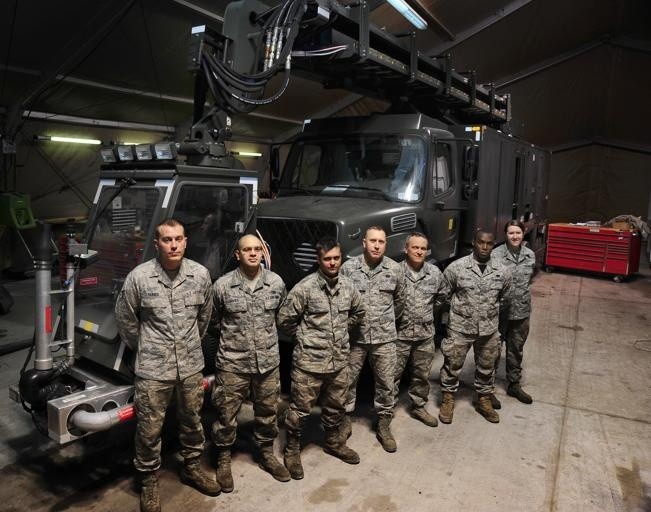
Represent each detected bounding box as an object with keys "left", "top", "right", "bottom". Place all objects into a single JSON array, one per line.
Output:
[
  {"left": 473, "top": 392, "right": 502, "bottom": 423},
  {"left": 139, "top": 471, "right": 162, "bottom": 512},
  {"left": 180, "top": 457, "right": 222, "bottom": 496},
  {"left": 372, "top": 414, "right": 398, "bottom": 453},
  {"left": 257, "top": 443, "right": 292, "bottom": 481},
  {"left": 284, "top": 433, "right": 305, "bottom": 480},
  {"left": 507, "top": 382, "right": 533, "bottom": 404},
  {"left": 324, "top": 428, "right": 361, "bottom": 464},
  {"left": 439, "top": 391, "right": 456, "bottom": 423},
  {"left": 344, "top": 414, "right": 353, "bottom": 441},
  {"left": 410, "top": 404, "right": 439, "bottom": 427},
  {"left": 215, "top": 446, "right": 235, "bottom": 493}
]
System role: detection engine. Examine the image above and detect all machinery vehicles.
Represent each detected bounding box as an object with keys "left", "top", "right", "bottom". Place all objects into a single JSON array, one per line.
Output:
[{"left": 4, "top": 2, "right": 551, "bottom": 445}]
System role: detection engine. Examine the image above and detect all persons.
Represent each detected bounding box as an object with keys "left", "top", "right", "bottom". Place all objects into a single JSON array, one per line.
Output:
[
  {"left": 112, "top": 217, "right": 222, "bottom": 512},
  {"left": 209, "top": 233, "right": 292, "bottom": 493},
  {"left": 276, "top": 235, "right": 366, "bottom": 480},
  {"left": 489, "top": 219, "right": 537, "bottom": 407},
  {"left": 344, "top": 225, "right": 407, "bottom": 452},
  {"left": 438, "top": 229, "right": 514, "bottom": 424},
  {"left": 200, "top": 214, "right": 224, "bottom": 281},
  {"left": 395, "top": 230, "right": 448, "bottom": 429}
]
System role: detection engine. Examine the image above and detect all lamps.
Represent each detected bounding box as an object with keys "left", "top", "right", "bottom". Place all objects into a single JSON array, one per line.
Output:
[
  {"left": 31, "top": 132, "right": 104, "bottom": 147},
  {"left": 228, "top": 150, "right": 263, "bottom": 158},
  {"left": 386, "top": 0, "right": 429, "bottom": 31},
  {"left": 108, "top": 140, "right": 143, "bottom": 148}
]
